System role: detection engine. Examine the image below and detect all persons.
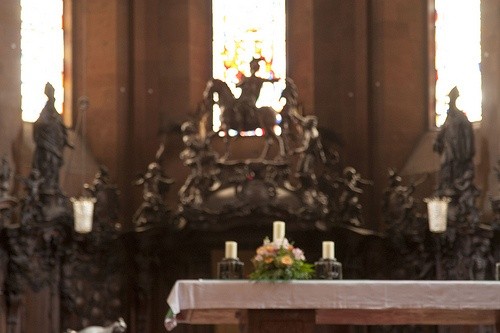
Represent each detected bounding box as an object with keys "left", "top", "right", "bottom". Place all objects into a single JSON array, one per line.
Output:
[{"left": 20, "top": 56, "right": 476, "bottom": 236}]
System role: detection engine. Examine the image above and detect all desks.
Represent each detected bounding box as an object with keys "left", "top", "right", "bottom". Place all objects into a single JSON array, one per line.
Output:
[{"left": 165, "top": 278, "right": 500, "bottom": 332}]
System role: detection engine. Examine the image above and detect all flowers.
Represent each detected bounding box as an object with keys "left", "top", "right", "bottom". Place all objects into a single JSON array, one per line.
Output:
[{"left": 247, "top": 235, "right": 319, "bottom": 283}]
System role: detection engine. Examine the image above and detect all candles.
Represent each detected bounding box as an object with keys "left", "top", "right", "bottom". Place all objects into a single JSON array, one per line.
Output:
[
  {"left": 225, "top": 240, "right": 238, "bottom": 259},
  {"left": 272, "top": 221, "right": 285, "bottom": 241},
  {"left": 322, "top": 241, "right": 335, "bottom": 259}
]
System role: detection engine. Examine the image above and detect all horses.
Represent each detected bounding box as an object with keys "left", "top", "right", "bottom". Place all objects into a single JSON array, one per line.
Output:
[{"left": 202, "top": 72, "right": 286, "bottom": 162}]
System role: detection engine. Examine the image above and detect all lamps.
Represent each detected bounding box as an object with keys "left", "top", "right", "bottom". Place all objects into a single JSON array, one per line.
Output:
[
  {"left": 423, "top": 128, "right": 451, "bottom": 231},
  {"left": 66, "top": 0, "right": 97, "bottom": 232}
]
